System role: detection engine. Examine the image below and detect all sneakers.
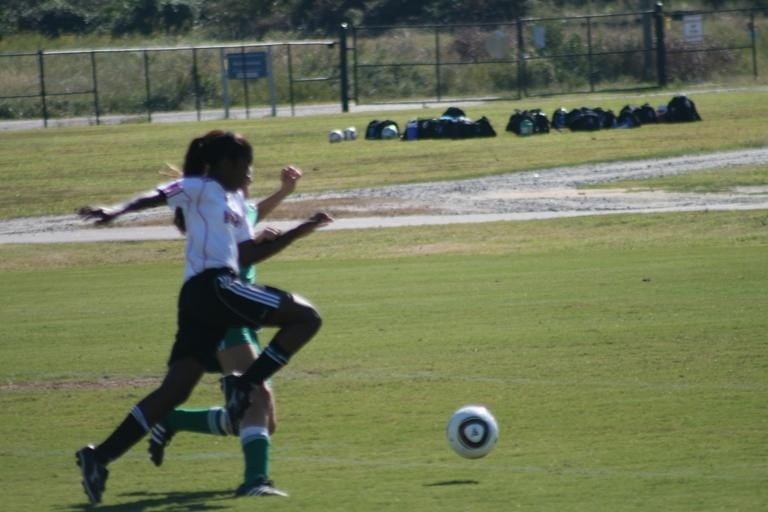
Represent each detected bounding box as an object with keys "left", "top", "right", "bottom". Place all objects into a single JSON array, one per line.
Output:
[
  {"left": 218, "top": 370, "right": 254, "bottom": 438},
  {"left": 147, "top": 420, "right": 172, "bottom": 467},
  {"left": 75, "top": 445, "right": 109, "bottom": 503},
  {"left": 238, "top": 480, "right": 287, "bottom": 496}
]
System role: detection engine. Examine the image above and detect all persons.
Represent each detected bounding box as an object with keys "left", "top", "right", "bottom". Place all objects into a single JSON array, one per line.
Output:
[
  {"left": 72, "top": 130, "right": 337, "bottom": 504},
  {"left": 145, "top": 128, "right": 303, "bottom": 500}
]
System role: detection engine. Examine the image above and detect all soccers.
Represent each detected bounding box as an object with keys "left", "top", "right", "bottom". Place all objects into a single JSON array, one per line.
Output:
[
  {"left": 344, "top": 126, "right": 357, "bottom": 140},
  {"left": 382, "top": 126, "right": 398, "bottom": 139},
  {"left": 328, "top": 130, "right": 343, "bottom": 142},
  {"left": 446, "top": 405, "right": 499, "bottom": 459}
]
